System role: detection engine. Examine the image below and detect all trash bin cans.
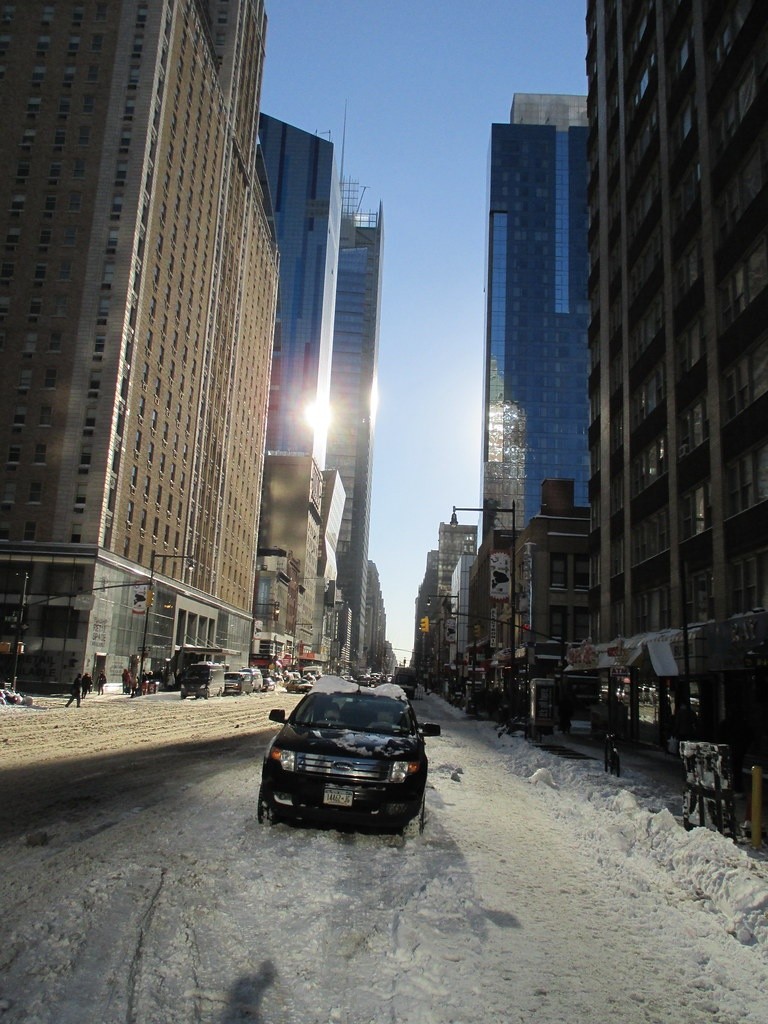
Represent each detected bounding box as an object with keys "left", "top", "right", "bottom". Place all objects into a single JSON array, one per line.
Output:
[
  {"left": 454, "top": 692, "right": 463, "bottom": 708},
  {"left": 679, "top": 741, "right": 737, "bottom": 842},
  {"left": 525, "top": 717, "right": 542, "bottom": 743},
  {"left": 467, "top": 697, "right": 477, "bottom": 714},
  {"left": 0, "top": 683, "right": 11, "bottom": 689},
  {"left": 147, "top": 679, "right": 156, "bottom": 694}
]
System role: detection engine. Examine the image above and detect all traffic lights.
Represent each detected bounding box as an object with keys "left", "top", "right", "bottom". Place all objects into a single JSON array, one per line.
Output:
[{"left": 420, "top": 616, "right": 429, "bottom": 633}]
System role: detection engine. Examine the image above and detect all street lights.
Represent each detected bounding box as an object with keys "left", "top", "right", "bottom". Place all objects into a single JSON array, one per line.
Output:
[
  {"left": 426, "top": 590, "right": 460, "bottom": 684},
  {"left": 247, "top": 602, "right": 280, "bottom": 669},
  {"left": 137, "top": 549, "right": 195, "bottom": 689}
]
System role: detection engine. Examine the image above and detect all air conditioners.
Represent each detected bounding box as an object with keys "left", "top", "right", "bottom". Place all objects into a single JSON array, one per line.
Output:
[{"left": 679, "top": 444, "right": 689, "bottom": 458}]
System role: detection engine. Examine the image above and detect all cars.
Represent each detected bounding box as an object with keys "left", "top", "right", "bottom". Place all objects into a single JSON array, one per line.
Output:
[
  {"left": 224, "top": 665, "right": 323, "bottom": 697},
  {"left": 257, "top": 676, "right": 442, "bottom": 838}
]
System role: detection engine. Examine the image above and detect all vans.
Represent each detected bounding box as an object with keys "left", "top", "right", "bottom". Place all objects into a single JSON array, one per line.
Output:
[{"left": 180, "top": 661, "right": 225, "bottom": 700}]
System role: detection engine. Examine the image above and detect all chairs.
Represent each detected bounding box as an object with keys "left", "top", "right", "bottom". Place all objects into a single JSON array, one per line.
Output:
[{"left": 316, "top": 702, "right": 347, "bottom": 725}]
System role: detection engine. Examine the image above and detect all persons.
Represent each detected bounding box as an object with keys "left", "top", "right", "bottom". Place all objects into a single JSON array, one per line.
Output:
[
  {"left": 143, "top": 668, "right": 163, "bottom": 681},
  {"left": 65, "top": 673, "right": 82, "bottom": 708},
  {"left": 718, "top": 704, "right": 754, "bottom": 798},
  {"left": 168, "top": 671, "right": 181, "bottom": 691},
  {"left": 121, "top": 669, "right": 130, "bottom": 685},
  {"left": 673, "top": 702, "right": 697, "bottom": 741},
  {"left": 558, "top": 694, "right": 573, "bottom": 734},
  {"left": 97, "top": 670, "right": 106, "bottom": 695},
  {"left": 81, "top": 672, "right": 91, "bottom": 699}
]
